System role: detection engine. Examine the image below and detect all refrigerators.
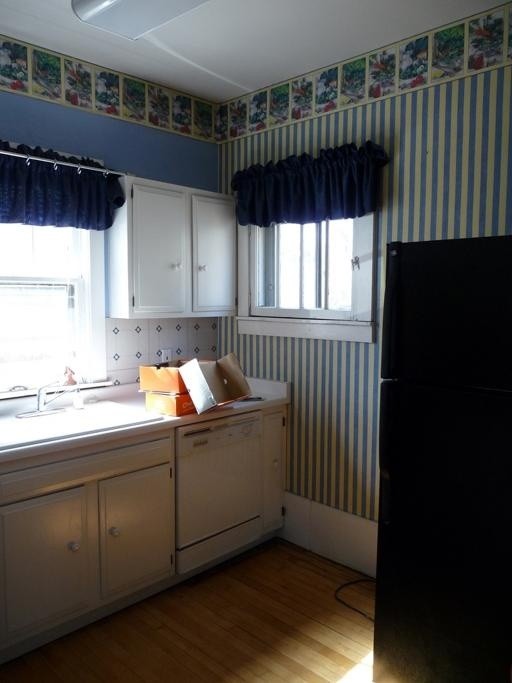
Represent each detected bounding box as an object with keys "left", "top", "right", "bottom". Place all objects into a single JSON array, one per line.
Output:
[{"left": 371, "top": 233, "right": 512, "bottom": 683}]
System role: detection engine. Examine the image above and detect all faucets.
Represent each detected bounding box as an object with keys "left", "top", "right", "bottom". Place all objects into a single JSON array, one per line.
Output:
[{"left": 16, "top": 380, "right": 81, "bottom": 418}]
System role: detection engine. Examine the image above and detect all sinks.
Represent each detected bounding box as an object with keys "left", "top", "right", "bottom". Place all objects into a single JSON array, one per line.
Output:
[{"left": 1, "top": 400, "right": 165, "bottom": 450}]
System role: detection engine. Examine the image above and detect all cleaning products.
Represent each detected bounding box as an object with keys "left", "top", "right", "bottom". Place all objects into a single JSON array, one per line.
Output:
[
  {"left": 73, "top": 380, "right": 84, "bottom": 409},
  {"left": 64, "top": 367, "right": 76, "bottom": 384}
]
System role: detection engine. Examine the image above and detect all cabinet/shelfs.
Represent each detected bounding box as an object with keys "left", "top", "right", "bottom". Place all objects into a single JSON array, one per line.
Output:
[
  {"left": 1, "top": 432, "right": 172, "bottom": 664},
  {"left": 108, "top": 184, "right": 234, "bottom": 321}
]
